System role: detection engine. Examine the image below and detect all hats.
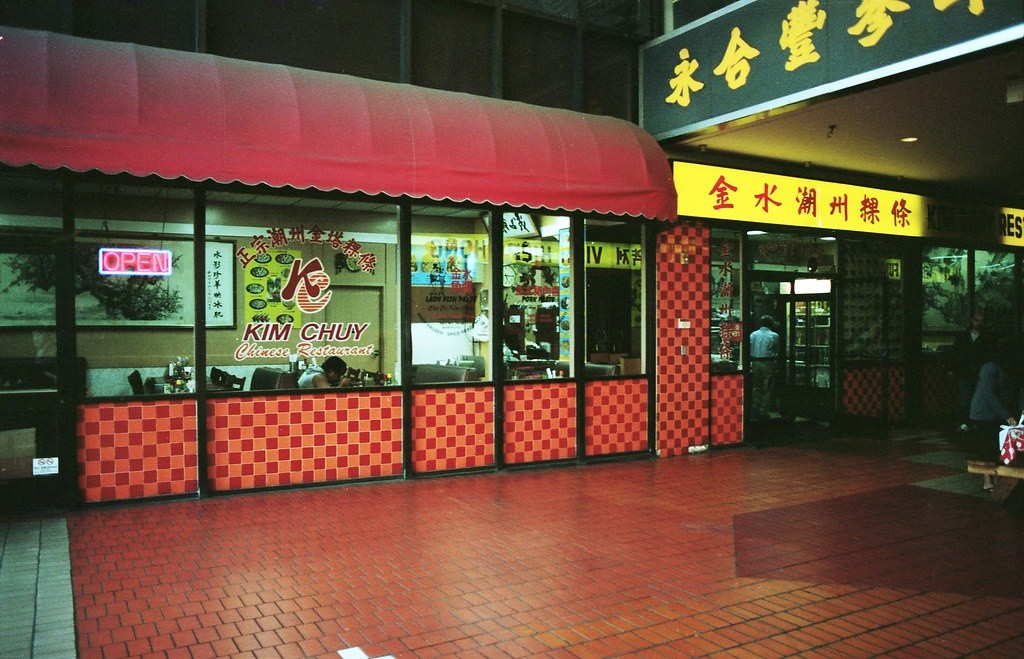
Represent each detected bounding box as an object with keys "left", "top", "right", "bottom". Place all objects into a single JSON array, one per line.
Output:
[{"left": 322, "top": 356, "right": 347, "bottom": 375}]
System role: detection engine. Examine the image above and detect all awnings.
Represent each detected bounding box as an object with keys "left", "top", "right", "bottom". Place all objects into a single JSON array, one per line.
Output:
[{"left": 0, "top": 26, "right": 678, "bottom": 222}]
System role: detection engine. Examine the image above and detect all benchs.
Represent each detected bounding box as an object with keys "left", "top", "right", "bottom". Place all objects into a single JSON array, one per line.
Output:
[
  {"left": 557, "top": 361, "right": 616, "bottom": 377},
  {"left": 414, "top": 364, "right": 479, "bottom": 382}
]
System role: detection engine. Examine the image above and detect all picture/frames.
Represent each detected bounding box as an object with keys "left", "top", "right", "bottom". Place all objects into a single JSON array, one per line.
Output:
[{"left": 0, "top": 226, "right": 239, "bottom": 331}]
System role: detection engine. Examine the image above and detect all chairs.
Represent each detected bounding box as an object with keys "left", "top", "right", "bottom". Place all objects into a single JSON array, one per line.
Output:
[
  {"left": 210, "top": 366, "right": 246, "bottom": 391},
  {"left": 347, "top": 366, "right": 382, "bottom": 385},
  {"left": 251, "top": 366, "right": 300, "bottom": 389},
  {"left": 127, "top": 369, "right": 155, "bottom": 394}
]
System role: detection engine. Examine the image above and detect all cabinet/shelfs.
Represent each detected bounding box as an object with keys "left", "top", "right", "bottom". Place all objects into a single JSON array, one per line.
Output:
[{"left": 784, "top": 302, "right": 830, "bottom": 388}]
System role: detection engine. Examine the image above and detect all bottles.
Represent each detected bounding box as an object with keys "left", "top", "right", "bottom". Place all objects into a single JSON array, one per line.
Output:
[
  {"left": 175, "top": 379, "right": 182, "bottom": 392},
  {"left": 387, "top": 374, "right": 392, "bottom": 384}
]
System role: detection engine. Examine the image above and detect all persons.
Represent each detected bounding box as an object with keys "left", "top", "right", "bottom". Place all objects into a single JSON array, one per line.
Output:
[
  {"left": 948, "top": 311, "right": 1018, "bottom": 492},
  {"left": 299, "top": 356, "right": 351, "bottom": 388},
  {"left": 750, "top": 315, "right": 780, "bottom": 418}
]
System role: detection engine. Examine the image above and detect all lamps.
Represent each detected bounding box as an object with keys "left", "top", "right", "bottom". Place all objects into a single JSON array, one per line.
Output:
[{"left": 807, "top": 257, "right": 818, "bottom": 274}]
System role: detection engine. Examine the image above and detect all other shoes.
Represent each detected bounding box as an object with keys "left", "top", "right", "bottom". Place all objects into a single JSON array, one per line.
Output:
[
  {"left": 767, "top": 412, "right": 782, "bottom": 420},
  {"left": 981, "top": 485, "right": 995, "bottom": 493},
  {"left": 750, "top": 422, "right": 768, "bottom": 430}
]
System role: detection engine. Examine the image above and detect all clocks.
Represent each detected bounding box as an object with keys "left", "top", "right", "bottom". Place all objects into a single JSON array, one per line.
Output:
[{"left": 503, "top": 264, "right": 518, "bottom": 288}]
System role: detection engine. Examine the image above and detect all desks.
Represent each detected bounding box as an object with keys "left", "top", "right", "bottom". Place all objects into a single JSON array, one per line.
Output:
[
  {"left": 154, "top": 376, "right": 227, "bottom": 393},
  {"left": 297, "top": 369, "right": 365, "bottom": 388}
]
